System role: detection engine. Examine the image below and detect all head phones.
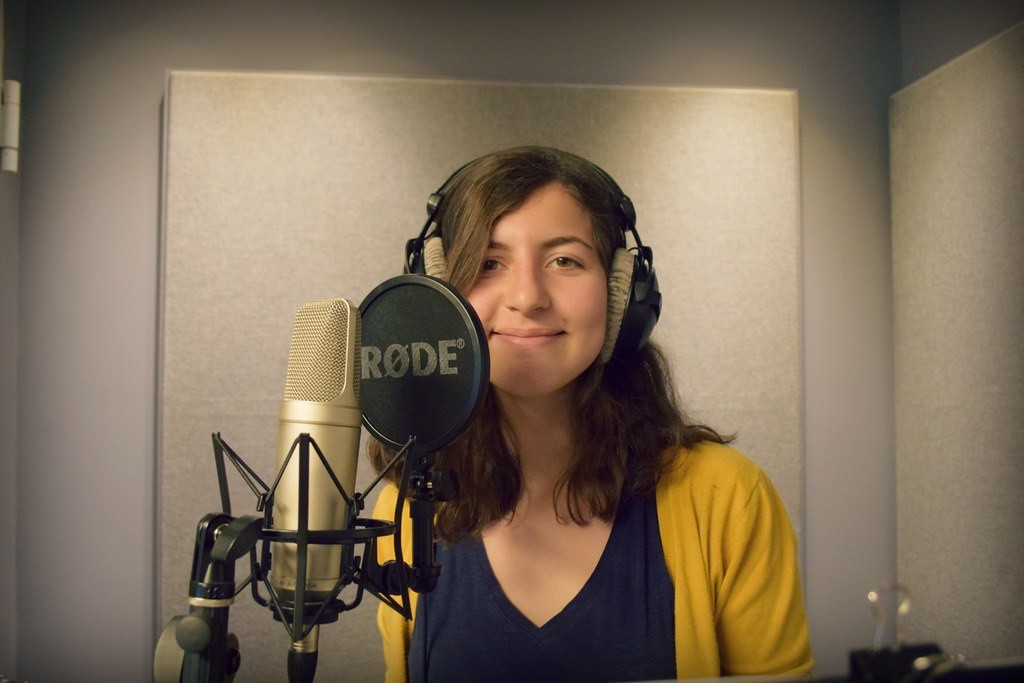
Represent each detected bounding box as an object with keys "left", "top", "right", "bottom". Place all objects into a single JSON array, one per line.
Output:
[{"left": 401, "top": 158, "right": 662, "bottom": 363}]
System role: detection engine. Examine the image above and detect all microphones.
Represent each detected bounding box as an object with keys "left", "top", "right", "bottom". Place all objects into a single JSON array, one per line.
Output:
[{"left": 267, "top": 298, "right": 365, "bottom": 683}]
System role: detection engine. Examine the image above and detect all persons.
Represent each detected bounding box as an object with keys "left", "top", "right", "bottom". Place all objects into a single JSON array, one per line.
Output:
[{"left": 369, "top": 145, "right": 819, "bottom": 683}]
